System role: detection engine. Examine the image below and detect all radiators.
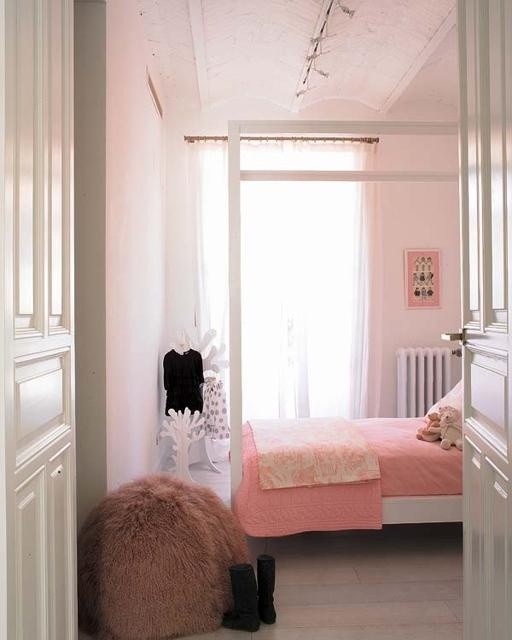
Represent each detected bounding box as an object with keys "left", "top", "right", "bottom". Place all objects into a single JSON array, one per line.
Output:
[{"left": 395, "top": 347, "right": 452, "bottom": 418}]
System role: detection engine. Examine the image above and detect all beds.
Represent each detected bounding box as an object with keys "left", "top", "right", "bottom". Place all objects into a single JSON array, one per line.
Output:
[{"left": 228, "top": 119, "right": 464, "bottom": 541}]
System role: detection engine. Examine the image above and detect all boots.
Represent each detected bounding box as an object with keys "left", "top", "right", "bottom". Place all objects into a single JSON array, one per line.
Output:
[
  {"left": 221, "top": 564, "right": 260, "bottom": 632},
  {"left": 256, "top": 555, "right": 276, "bottom": 624}
]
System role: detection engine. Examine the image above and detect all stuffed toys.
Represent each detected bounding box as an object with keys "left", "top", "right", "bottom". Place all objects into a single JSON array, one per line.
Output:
[
  {"left": 437, "top": 405, "right": 462, "bottom": 450},
  {"left": 416, "top": 411, "right": 440, "bottom": 441}
]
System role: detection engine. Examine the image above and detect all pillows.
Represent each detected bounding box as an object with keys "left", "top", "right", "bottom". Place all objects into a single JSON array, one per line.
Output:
[{"left": 424, "top": 378, "right": 463, "bottom": 428}]
